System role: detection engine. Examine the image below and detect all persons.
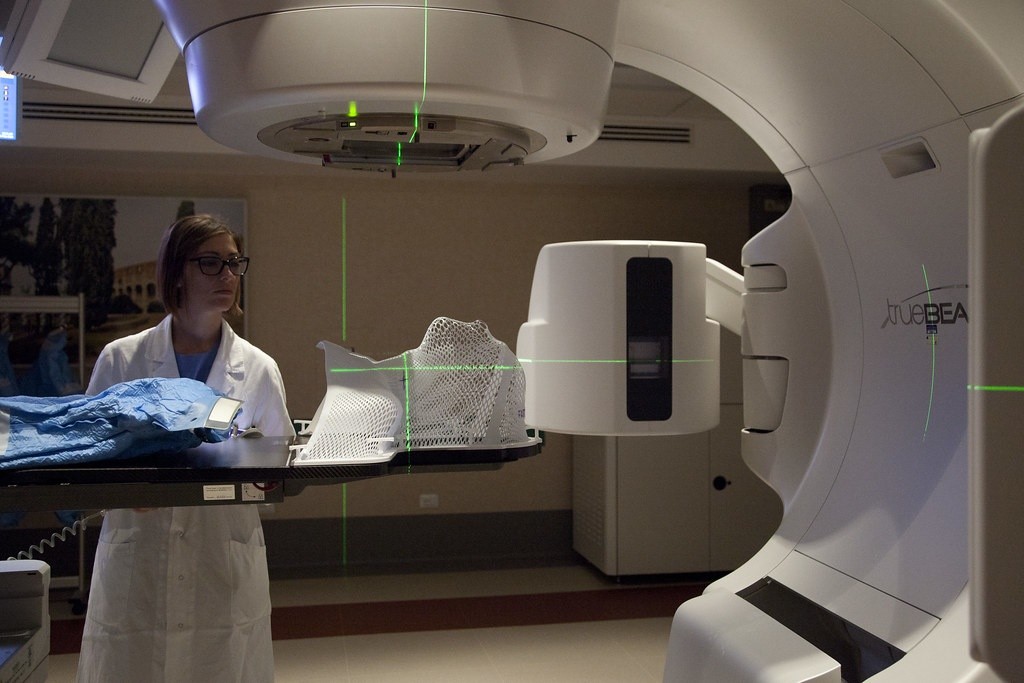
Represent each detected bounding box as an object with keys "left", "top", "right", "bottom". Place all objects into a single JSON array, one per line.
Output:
[{"left": 78, "top": 213, "right": 296, "bottom": 683}]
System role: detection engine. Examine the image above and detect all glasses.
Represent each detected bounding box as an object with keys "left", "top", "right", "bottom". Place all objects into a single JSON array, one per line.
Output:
[{"left": 186, "top": 256, "right": 250, "bottom": 276}]
